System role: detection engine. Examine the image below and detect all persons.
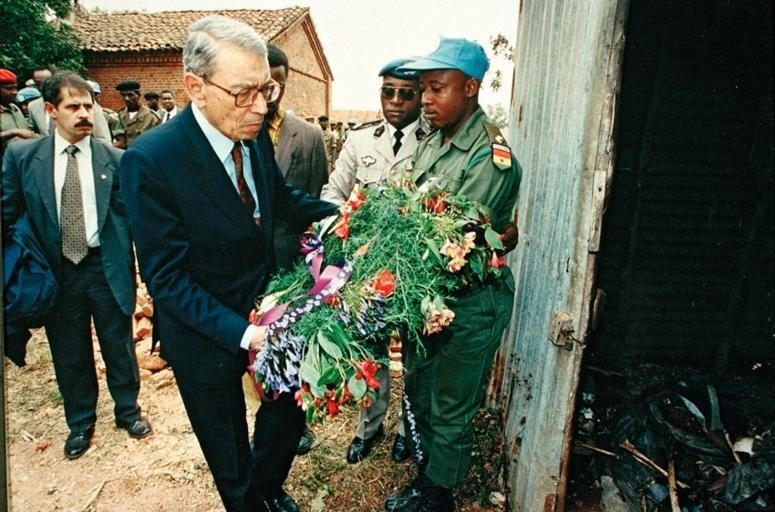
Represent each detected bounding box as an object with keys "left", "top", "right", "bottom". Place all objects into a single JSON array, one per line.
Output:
[
  {"left": 2, "top": 68, "right": 112, "bottom": 154},
  {"left": 3, "top": 71, "right": 151, "bottom": 459},
  {"left": 384, "top": 36, "right": 522, "bottom": 510},
  {"left": 120, "top": 15, "right": 347, "bottom": 512},
  {"left": 306, "top": 114, "right": 358, "bottom": 172},
  {"left": 86, "top": 79, "right": 182, "bottom": 149},
  {"left": 267, "top": 43, "right": 330, "bottom": 453},
  {"left": 320, "top": 58, "right": 430, "bottom": 463}
]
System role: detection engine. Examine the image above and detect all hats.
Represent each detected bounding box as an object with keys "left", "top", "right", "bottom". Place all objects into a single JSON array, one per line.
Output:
[
  {"left": 378, "top": 56, "right": 420, "bottom": 81},
  {"left": 395, "top": 38, "right": 490, "bottom": 84},
  {"left": 0, "top": 68, "right": 161, "bottom": 104}
]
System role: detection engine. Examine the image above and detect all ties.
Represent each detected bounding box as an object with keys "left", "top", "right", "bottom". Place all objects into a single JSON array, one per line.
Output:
[
  {"left": 392, "top": 130, "right": 404, "bottom": 156},
  {"left": 230, "top": 140, "right": 255, "bottom": 218},
  {"left": 60, "top": 145, "right": 88, "bottom": 265},
  {"left": 166, "top": 113, "right": 170, "bottom": 121}
]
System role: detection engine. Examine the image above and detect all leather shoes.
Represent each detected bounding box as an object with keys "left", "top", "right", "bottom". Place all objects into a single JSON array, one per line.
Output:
[
  {"left": 116, "top": 417, "right": 151, "bottom": 438},
  {"left": 392, "top": 433, "right": 411, "bottom": 462},
  {"left": 346, "top": 423, "right": 384, "bottom": 464},
  {"left": 297, "top": 426, "right": 311, "bottom": 455},
  {"left": 384, "top": 473, "right": 454, "bottom": 511},
  {"left": 64, "top": 423, "right": 94, "bottom": 460},
  {"left": 270, "top": 487, "right": 299, "bottom": 512}
]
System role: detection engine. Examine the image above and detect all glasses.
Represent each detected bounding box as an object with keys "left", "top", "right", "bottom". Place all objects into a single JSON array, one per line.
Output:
[
  {"left": 379, "top": 87, "right": 420, "bottom": 101},
  {"left": 204, "top": 78, "right": 281, "bottom": 108}
]
